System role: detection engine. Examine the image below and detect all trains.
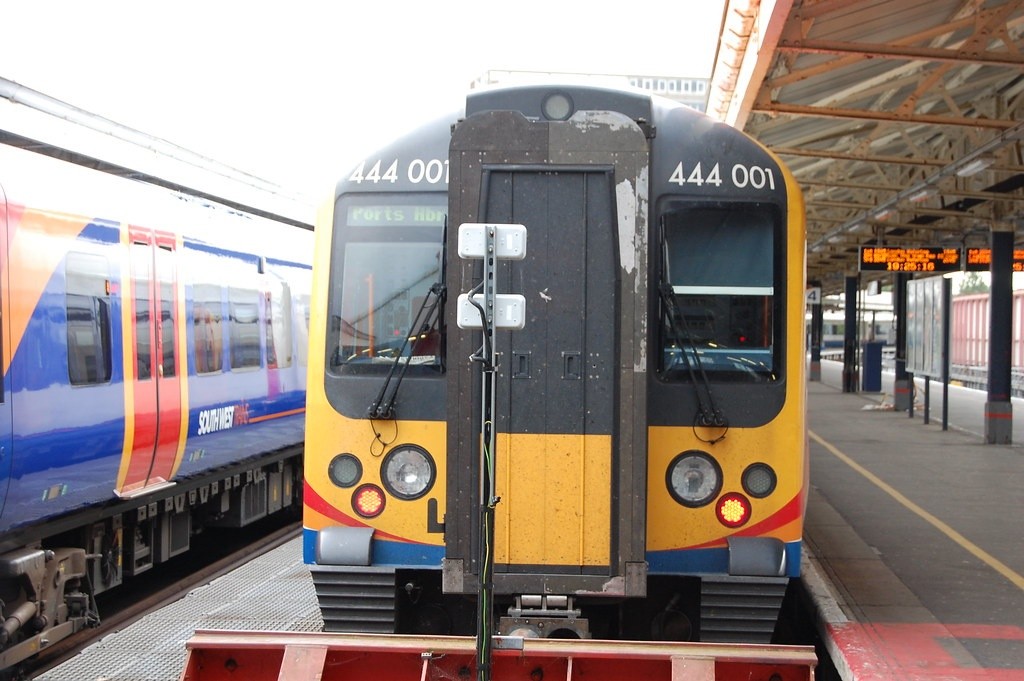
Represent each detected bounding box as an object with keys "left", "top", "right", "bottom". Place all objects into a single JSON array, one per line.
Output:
[
  {"left": 297, "top": 73, "right": 812, "bottom": 652},
  {"left": 0, "top": 68, "right": 321, "bottom": 675}
]
